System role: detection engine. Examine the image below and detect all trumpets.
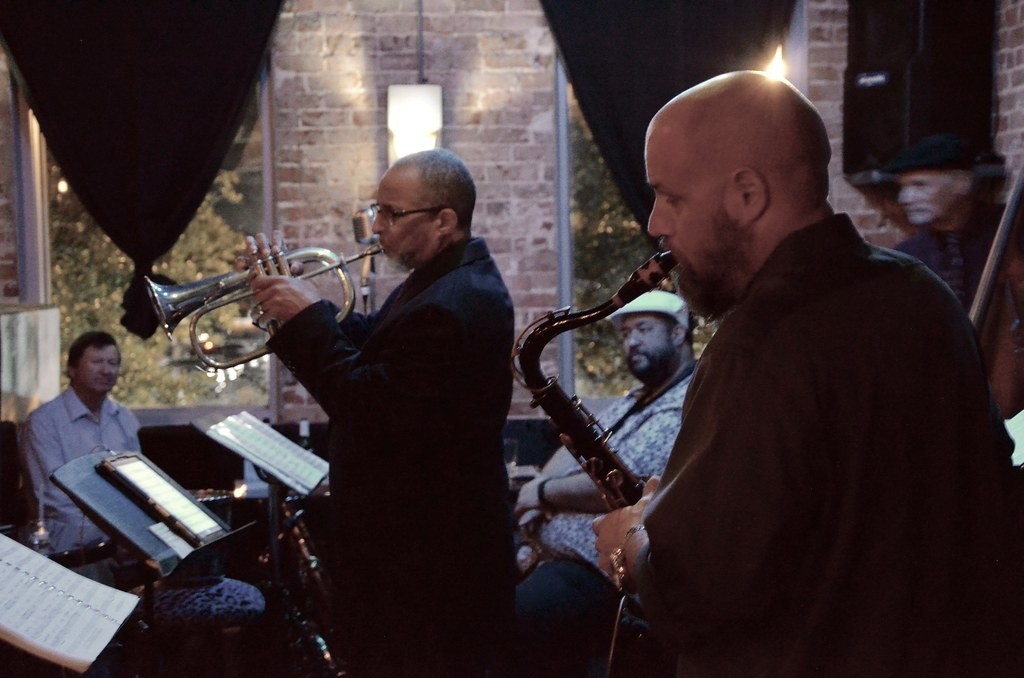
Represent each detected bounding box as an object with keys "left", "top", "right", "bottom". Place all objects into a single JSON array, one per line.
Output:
[{"left": 142, "top": 244, "right": 382, "bottom": 368}]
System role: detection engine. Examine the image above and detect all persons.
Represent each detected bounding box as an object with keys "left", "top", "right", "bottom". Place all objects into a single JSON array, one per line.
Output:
[
  {"left": 23, "top": 331, "right": 140, "bottom": 555},
  {"left": 516, "top": 289, "right": 697, "bottom": 678},
  {"left": 243, "top": 152, "right": 521, "bottom": 678},
  {"left": 592, "top": 68, "right": 1024, "bottom": 678}
]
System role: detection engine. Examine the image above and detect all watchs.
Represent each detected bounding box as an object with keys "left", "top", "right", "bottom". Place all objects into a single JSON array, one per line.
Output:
[{"left": 608, "top": 524, "right": 646, "bottom": 591}]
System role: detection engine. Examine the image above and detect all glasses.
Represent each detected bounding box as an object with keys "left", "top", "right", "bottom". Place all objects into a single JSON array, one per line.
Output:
[{"left": 371, "top": 203, "right": 442, "bottom": 226}]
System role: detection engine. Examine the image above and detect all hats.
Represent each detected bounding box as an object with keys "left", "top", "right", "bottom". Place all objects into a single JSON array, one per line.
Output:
[
  {"left": 884, "top": 134, "right": 978, "bottom": 170},
  {"left": 605, "top": 290, "right": 690, "bottom": 329}
]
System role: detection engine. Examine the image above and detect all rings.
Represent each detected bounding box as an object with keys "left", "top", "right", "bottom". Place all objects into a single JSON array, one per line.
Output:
[{"left": 256, "top": 305, "right": 264, "bottom": 316}]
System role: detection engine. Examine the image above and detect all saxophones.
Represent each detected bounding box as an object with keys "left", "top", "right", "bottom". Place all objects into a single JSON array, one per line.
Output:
[{"left": 515, "top": 254, "right": 679, "bottom": 525}]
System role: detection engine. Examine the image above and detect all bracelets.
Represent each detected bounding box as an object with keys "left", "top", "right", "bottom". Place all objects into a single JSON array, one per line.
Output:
[{"left": 538, "top": 475, "right": 553, "bottom": 510}]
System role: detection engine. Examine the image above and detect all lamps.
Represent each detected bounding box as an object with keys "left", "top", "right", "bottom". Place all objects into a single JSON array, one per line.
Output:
[{"left": 388, "top": 84, "right": 443, "bottom": 168}]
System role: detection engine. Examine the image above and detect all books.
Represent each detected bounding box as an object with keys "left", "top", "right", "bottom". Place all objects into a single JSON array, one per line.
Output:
[{"left": 207, "top": 410, "right": 330, "bottom": 492}]
